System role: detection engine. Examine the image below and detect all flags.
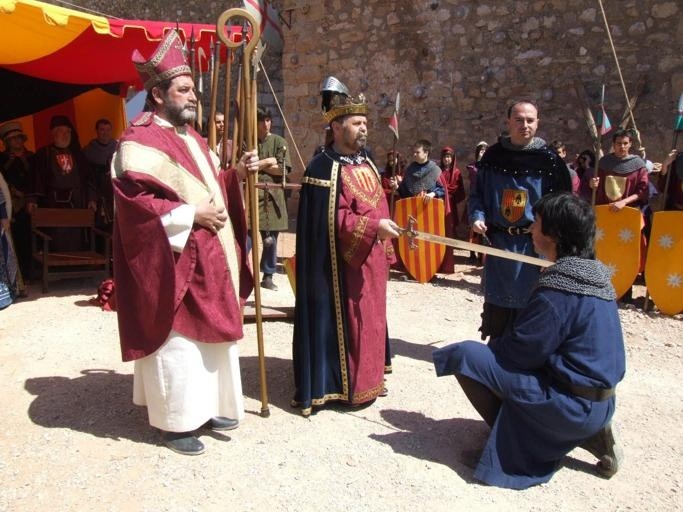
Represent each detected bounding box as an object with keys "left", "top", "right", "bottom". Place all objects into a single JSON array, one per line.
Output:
[
  {"left": 598, "top": 106, "right": 611, "bottom": 135},
  {"left": 242, "top": 1, "right": 286, "bottom": 55},
  {"left": 388, "top": 113, "right": 399, "bottom": 139}
]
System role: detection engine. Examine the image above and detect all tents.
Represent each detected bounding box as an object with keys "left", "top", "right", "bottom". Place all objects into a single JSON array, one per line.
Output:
[{"left": 0, "top": 0, "right": 260, "bottom": 278}]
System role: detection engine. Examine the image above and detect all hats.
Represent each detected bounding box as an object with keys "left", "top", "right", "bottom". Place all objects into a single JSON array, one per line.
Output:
[
  {"left": 385, "top": 150, "right": 407, "bottom": 177},
  {"left": 49, "top": 114, "right": 82, "bottom": 159},
  {"left": 130, "top": 27, "right": 192, "bottom": 92},
  {"left": 440, "top": 147, "right": 454, "bottom": 158},
  {"left": 0, "top": 121, "right": 28, "bottom": 143},
  {"left": 317, "top": 74, "right": 368, "bottom": 128}
]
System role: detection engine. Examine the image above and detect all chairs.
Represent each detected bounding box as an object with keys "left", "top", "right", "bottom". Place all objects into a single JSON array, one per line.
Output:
[{"left": 30, "top": 208, "right": 111, "bottom": 294}]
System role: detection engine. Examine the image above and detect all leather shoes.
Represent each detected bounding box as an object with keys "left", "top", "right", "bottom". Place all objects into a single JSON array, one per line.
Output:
[
  {"left": 202, "top": 415, "right": 239, "bottom": 431},
  {"left": 457, "top": 447, "right": 484, "bottom": 468},
  {"left": 538, "top": 364, "right": 618, "bottom": 400},
  {"left": 262, "top": 277, "right": 278, "bottom": 291},
  {"left": 156, "top": 428, "right": 208, "bottom": 455},
  {"left": 595, "top": 420, "right": 625, "bottom": 478}
]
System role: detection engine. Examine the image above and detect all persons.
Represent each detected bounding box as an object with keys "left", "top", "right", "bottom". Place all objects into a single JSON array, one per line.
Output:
[
  {"left": 384, "top": 148, "right": 406, "bottom": 196},
  {"left": 291, "top": 76, "right": 400, "bottom": 414},
  {"left": 549, "top": 140, "right": 579, "bottom": 193},
  {"left": 657, "top": 149, "right": 683, "bottom": 212},
  {"left": 1, "top": 121, "right": 32, "bottom": 169},
  {"left": 82, "top": 119, "right": 119, "bottom": 225},
  {"left": 577, "top": 150, "right": 596, "bottom": 194},
  {"left": 27, "top": 114, "right": 97, "bottom": 251},
  {"left": 467, "top": 141, "right": 490, "bottom": 266},
  {"left": 433, "top": 190, "right": 626, "bottom": 490},
  {"left": 591, "top": 130, "right": 648, "bottom": 299},
  {"left": 110, "top": 29, "right": 259, "bottom": 455},
  {"left": 440, "top": 146, "right": 465, "bottom": 225},
  {"left": 396, "top": 139, "right": 454, "bottom": 275},
  {"left": 0, "top": 172, "right": 28, "bottom": 308},
  {"left": 248, "top": 106, "right": 292, "bottom": 291},
  {"left": 203, "top": 110, "right": 237, "bottom": 164},
  {"left": 470, "top": 100, "right": 572, "bottom": 342}
]
